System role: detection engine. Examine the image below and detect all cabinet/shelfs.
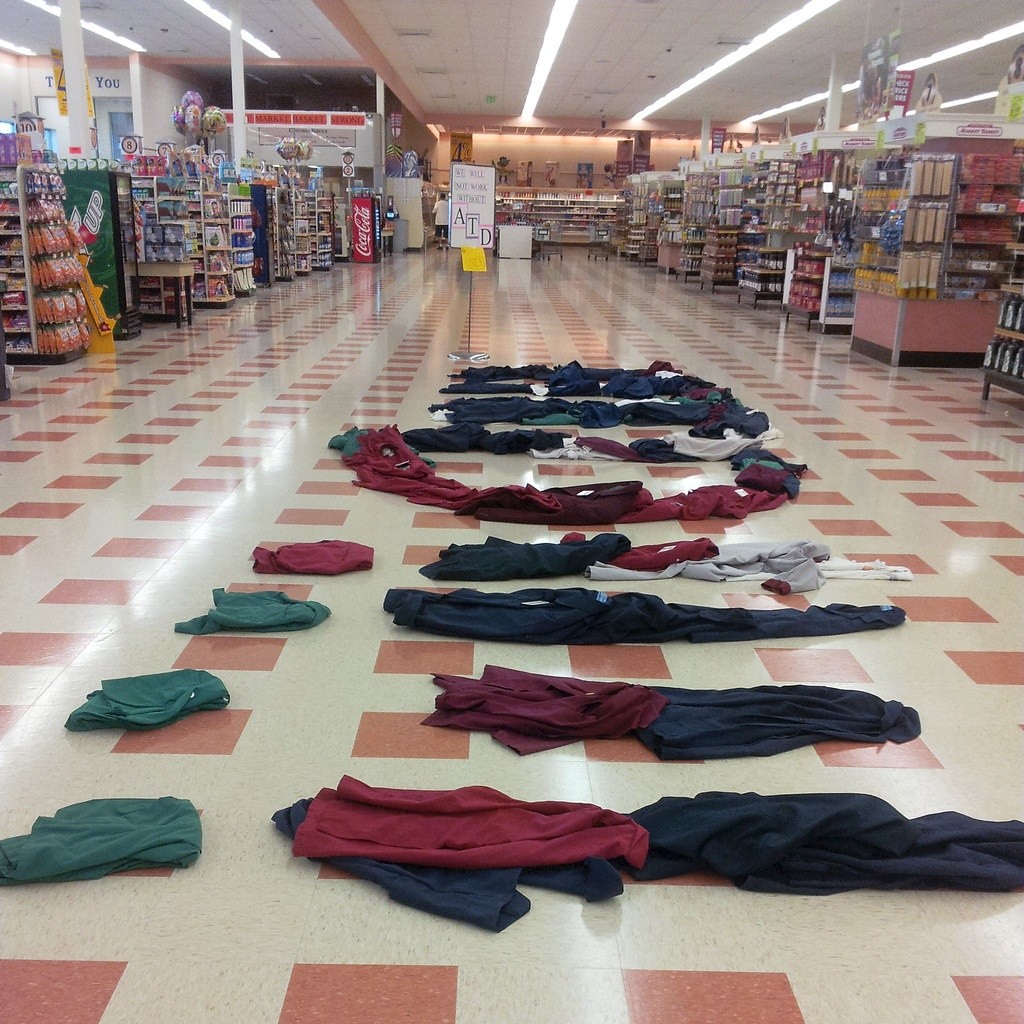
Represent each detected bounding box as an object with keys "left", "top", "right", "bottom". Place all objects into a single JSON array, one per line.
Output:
[
  {"left": 622, "top": 104, "right": 1024, "bottom": 396},
  {"left": 386, "top": 178, "right": 625, "bottom": 245},
  {"left": 0, "top": 153, "right": 384, "bottom": 404}
]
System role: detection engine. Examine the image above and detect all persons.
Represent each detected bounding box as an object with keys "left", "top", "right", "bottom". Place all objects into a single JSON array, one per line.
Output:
[
  {"left": 299, "top": 205, "right": 307, "bottom": 216},
  {"left": 210, "top": 202, "right": 222, "bottom": 219},
  {"left": 432, "top": 193, "right": 451, "bottom": 251},
  {"left": 212, "top": 281, "right": 225, "bottom": 298}
]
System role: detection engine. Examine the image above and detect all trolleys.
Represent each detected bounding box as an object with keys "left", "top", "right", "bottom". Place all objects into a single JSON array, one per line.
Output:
[
  {"left": 535, "top": 222, "right": 563, "bottom": 262},
  {"left": 587, "top": 221, "right": 612, "bottom": 262}
]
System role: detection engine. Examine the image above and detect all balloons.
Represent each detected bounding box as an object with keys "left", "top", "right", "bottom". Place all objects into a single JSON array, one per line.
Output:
[
  {"left": 275, "top": 136, "right": 313, "bottom": 164},
  {"left": 172, "top": 91, "right": 227, "bottom": 139}
]
